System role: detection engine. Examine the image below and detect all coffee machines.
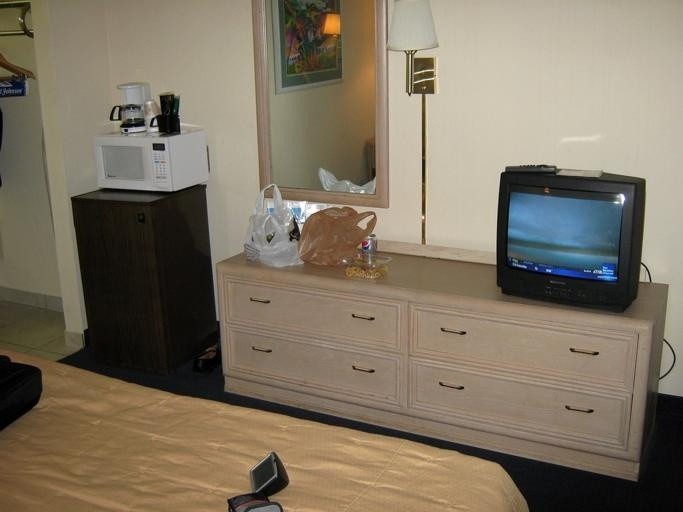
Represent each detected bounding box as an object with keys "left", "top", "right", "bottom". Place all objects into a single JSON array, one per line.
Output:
[{"left": 107, "top": 82, "right": 149, "bottom": 133}]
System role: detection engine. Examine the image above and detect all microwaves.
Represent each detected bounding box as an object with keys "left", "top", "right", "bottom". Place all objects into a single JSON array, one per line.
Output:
[{"left": 94, "top": 123, "right": 211, "bottom": 191}]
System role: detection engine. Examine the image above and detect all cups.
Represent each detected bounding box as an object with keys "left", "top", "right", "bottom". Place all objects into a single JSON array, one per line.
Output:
[
  {"left": 146, "top": 100, "right": 160, "bottom": 132},
  {"left": 158, "top": 93, "right": 184, "bottom": 133}
]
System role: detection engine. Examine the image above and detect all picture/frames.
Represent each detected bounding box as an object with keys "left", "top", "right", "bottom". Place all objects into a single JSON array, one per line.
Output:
[{"left": 272, "top": 0, "right": 344, "bottom": 95}]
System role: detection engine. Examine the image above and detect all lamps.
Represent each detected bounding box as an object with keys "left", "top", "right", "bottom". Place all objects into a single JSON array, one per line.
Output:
[
  {"left": 385, "top": 1, "right": 437, "bottom": 95},
  {"left": 324, "top": 13, "right": 341, "bottom": 39}
]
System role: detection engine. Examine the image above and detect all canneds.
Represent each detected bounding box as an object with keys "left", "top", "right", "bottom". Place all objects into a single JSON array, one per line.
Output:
[{"left": 362, "top": 234, "right": 378, "bottom": 255}]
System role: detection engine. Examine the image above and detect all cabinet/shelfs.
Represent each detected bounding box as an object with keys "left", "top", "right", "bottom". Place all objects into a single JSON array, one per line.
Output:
[{"left": 217, "top": 245, "right": 671, "bottom": 483}]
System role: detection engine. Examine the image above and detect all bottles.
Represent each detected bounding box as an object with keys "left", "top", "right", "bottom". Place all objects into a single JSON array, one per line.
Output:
[
  {"left": 287, "top": 206, "right": 304, "bottom": 242},
  {"left": 264, "top": 206, "right": 281, "bottom": 242}
]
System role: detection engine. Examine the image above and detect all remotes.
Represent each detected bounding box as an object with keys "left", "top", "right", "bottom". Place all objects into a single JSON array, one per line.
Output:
[{"left": 506, "top": 165, "right": 557, "bottom": 173}]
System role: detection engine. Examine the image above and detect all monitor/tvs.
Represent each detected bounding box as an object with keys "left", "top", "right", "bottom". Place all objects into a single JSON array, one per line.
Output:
[{"left": 498, "top": 170, "right": 645, "bottom": 312}]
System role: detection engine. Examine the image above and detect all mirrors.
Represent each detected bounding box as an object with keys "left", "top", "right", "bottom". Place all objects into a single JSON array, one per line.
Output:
[{"left": 250, "top": 0, "right": 389, "bottom": 209}]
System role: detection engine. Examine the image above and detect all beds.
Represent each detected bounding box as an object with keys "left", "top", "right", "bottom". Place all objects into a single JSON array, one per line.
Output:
[{"left": 0, "top": 351, "right": 530, "bottom": 511}]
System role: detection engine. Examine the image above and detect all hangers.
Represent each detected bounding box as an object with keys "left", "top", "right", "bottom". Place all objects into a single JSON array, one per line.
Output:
[{"left": 0, "top": 53, "right": 34, "bottom": 82}]
